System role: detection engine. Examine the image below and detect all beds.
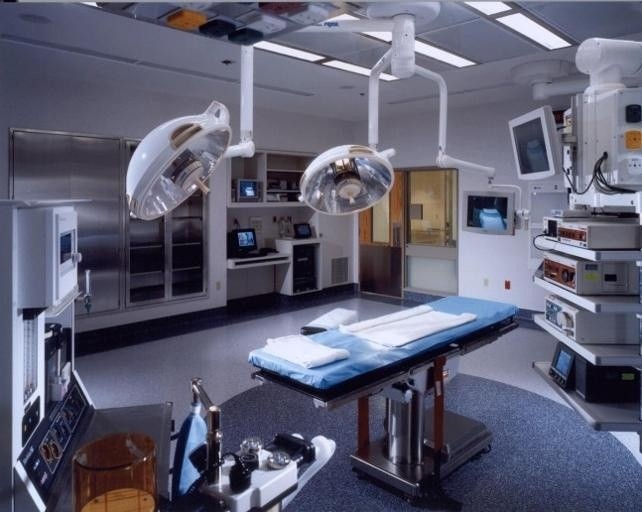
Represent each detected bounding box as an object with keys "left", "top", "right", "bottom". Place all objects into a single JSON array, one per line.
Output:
[{"left": 245, "top": 294, "right": 521, "bottom": 502}]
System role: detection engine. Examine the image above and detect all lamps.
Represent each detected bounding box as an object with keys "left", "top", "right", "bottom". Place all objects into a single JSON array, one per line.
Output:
[
  {"left": 124, "top": 97, "right": 234, "bottom": 221},
  {"left": 298, "top": 146, "right": 396, "bottom": 218}
]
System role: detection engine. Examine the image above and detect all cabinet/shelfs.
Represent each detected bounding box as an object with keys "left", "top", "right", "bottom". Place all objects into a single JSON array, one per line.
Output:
[
  {"left": 227, "top": 141, "right": 317, "bottom": 208},
  {"left": 126, "top": 137, "right": 211, "bottom": 310}
]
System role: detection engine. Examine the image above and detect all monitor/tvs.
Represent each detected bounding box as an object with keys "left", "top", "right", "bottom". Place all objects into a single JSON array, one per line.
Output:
[
  {"left": 460, "top": 190, "right": 516, "bottom": 236},
  {"left": 236, "top": 179, "right": 260, "bottom": 202},
  {"left": 508, "top": 104, "right": 561, "bottom": 182},
  {"left": 227, "top": 228, "right": 257, "bottom": 252}
]
